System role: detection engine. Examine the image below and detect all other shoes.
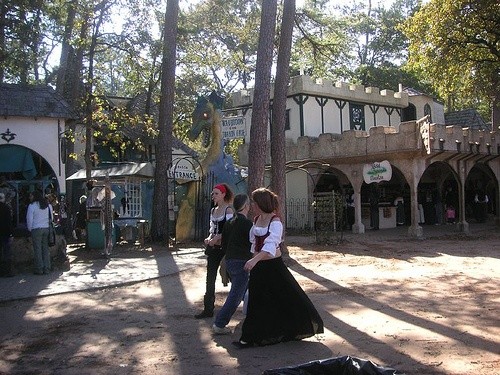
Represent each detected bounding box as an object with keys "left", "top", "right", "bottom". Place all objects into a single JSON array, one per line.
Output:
[
  {"left": 34, "top": 269, "right": 49, "bottom": 274},
  {"left": 212, "top": 324, "right": 231, "bottom": 335},
  {"left": 1, "top": 273, "right": 14, "bottom": 277},
  {"left": 232, "top": 341, "right": 252, "bottom": 347}
]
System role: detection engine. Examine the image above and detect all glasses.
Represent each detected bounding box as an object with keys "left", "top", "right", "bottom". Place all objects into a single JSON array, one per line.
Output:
[{"left": 211, "top": 192, "right": 218, "bottom": 196}]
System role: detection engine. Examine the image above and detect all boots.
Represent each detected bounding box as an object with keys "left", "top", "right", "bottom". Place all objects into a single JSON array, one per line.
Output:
[{"left": 194, "top": 295, "right": 215, "bottom": 318}]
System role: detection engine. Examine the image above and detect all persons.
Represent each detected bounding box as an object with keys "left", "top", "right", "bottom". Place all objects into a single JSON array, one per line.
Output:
[
  {"left": 211, "top": 194, "right": 253, "bottom": 335},
  {"left": 0, "top": 188, "right": 16, "bottom": 278},
  {"left": 370, "top": 186, "right": 381, "bottom": 230},
  {"left": 445, "top": 184, "right": 458, "bottom": 225},
  {"left": 26, "top": 190, "right": 54, "bottom": 276},
  {"left": 430, "top": 189, "right": 443, "bottom": 226},
  {"left": 341, "top": 192, "right": 356, "bottom": 231},
  {"left": 475, "top": 185, "right": 490, "bottom": 223},
  {"left": 394, "top": 192, "right": 406, "bottom": 226},
  {"left": 59, "top": 194, "right": 69, "bottom": 240},
  {"left": 73, "top": 194, "right": 88, "bottom": 239},
  {"left": 196, "top": 184, "right": 234, "bottom": 318},
  {"left": 233, "top": 187, "right": 324, "bottom": 349}
]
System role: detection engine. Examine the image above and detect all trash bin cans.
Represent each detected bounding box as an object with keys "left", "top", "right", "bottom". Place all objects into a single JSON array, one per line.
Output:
[
  {"left": 85, "top": 204, "right": 120, "bottom": 250},
  {"left": 125, "top": 225, "right": 136, "bottom": 244}
]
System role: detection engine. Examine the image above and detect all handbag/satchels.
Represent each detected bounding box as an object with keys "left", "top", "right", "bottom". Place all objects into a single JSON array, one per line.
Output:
[{"left": 48, "top": 206, "right": 56, "bottom": 246}]
